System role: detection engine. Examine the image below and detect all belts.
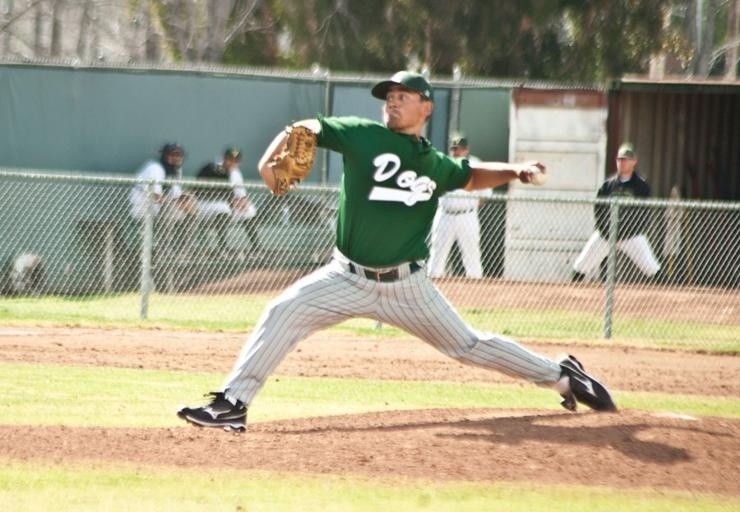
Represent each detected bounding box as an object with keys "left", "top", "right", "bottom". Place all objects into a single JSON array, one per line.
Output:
[
  {"left": 446, "top": 209, "right": 473, "bottom": 214},
  {"left": 348, "top": 263, "right": 420, "bottom": 283}
]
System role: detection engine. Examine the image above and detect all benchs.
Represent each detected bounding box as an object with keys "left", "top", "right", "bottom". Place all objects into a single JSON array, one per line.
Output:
[{"left": 70, "top": 208, "right": 344, "bottom": 300}]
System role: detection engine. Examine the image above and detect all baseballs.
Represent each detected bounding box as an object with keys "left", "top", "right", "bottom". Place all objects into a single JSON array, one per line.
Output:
[{"left": 528, "top": 166, "right": 546, "bottom": 184}]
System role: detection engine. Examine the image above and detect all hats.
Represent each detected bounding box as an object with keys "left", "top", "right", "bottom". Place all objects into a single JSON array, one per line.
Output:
[
  {"left": 223, "top": 149, "right": 242, "bottom": 161},
  {"left": 371, "top": 70, "right": 435, "bottom": 101},
  {"left": 450, "top": 135, "right": 468, "bottom": 149},
  {"left": 617, "top": 143, "right": 636, "bottom": 159}
]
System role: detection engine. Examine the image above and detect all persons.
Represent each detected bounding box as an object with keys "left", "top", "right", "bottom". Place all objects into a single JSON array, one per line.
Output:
[
  {"left": 188, "top": 149, "right": 268, "bottom": 260},
  {"left": 571, "top": 146, "right": 668, "bottom": 284},
  {"left": 177, "top": 71, "right": 617, "bottom": 433},
  {"left": 425, "top": 137, "right": 493, "bottom": 278},
  {"left": 129, "top": 144, "right": 199, "bottom": 259}
]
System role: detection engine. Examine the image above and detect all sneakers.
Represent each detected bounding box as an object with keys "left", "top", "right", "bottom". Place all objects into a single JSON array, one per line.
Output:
[
  {"left": 177, "top": 389, "right": 248, "bottom": 433},
  {"left": 557, "top": 356, "right": 615, "bottom": 412}
]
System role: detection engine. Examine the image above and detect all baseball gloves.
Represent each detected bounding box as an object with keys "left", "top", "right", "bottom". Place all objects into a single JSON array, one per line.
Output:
[{"left": 271, "top": 125, "right": 316, "bottom": 194}]
